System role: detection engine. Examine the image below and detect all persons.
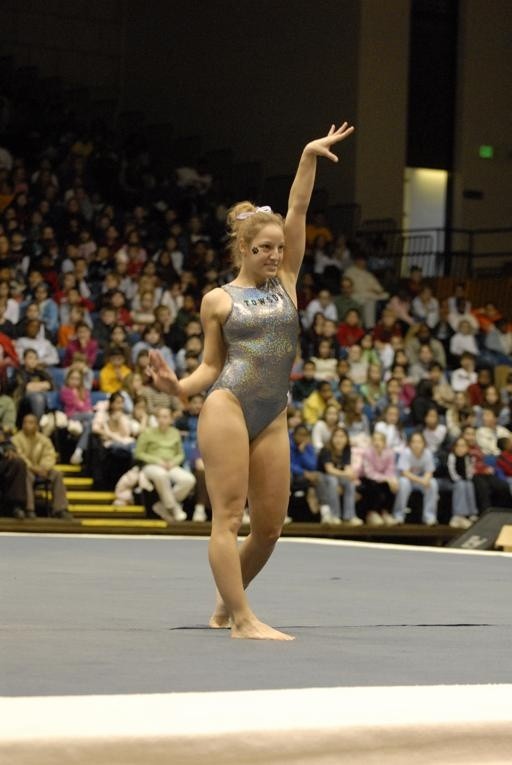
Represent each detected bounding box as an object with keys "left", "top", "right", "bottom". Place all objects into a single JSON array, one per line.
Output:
[
  {"left": 149, "top": 121, "right": 355, "bottom": 640},
  {"left": 0, "top": 129, "right": 512, "bottom": 526}
]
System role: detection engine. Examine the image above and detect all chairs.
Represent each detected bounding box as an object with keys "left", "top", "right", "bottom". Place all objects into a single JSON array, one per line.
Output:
[
  {"left": 34, "top": 475, "right": 51, "bottom": 517},
  {"left": 141, "top": 461, "right": 453, "bottom": 520}
]
